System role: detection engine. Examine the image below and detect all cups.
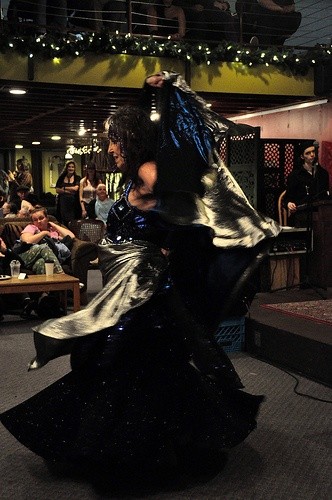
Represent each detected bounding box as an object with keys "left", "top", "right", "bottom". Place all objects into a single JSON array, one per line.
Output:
[
  {"left": 44, "top": 260, "right": 54, "bottom": 277},
  {"left": 10, "top": 264, "right": 21, "bottom": 277}
]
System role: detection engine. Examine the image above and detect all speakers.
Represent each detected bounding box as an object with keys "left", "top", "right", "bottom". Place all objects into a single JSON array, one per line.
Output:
[{"left": 314, "top": 61, "right": 332, "bottom": 95}]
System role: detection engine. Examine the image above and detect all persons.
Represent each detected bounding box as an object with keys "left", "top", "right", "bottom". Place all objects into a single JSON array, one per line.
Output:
[
  {"left": 284, "top": 142, "right": 330, "bottom": 286},
  {"left": 0, "top": 70, "right": 283, "bottom": 500},
  {"left": 0, "top": 159, "right": 118, "bottom": 318},
  {"left": 7, "top": 0, "right": 302, "bottom": 45}
]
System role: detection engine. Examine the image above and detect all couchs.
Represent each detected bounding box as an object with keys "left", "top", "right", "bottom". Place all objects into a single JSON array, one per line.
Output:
[{"left": 0, "top": 215, "right": 99, "bottom": 306}]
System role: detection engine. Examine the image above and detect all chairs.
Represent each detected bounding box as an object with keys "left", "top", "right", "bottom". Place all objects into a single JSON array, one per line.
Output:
[{"left": 74, "top": 219, "right": 105, "bottom": 242}]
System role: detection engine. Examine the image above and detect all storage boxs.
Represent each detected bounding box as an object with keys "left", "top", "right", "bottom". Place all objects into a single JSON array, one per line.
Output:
[{"left": 215, "top": 315, "right": 247, "bottom": 353}]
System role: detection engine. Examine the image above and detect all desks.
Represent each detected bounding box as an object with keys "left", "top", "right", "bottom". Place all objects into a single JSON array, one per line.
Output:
[{"left": 0, "top": 273, "right": 80, "bottom": 314}]
